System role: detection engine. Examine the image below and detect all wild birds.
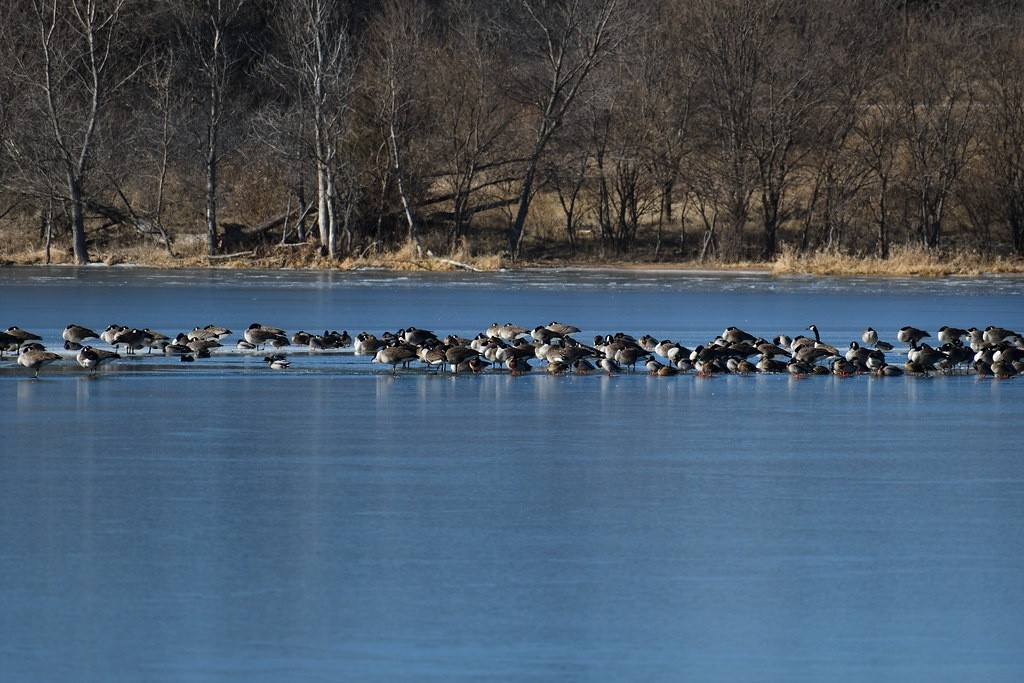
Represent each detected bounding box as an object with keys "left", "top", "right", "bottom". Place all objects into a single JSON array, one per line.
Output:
[
  {"left": 261, "top": 354, "right": 292, "bottom": 371},
  {"left": 236, "top": 323, "right": 352, "bottom": 352},
  {"left": 61, "top": 323, "right": 234, "bottom": 378},
  {"left": 353, "top": 321, "right": 1024, "bottom": 379},
  {"left": 0, "top": 326, "right": 63, "bottom": 379}
]
